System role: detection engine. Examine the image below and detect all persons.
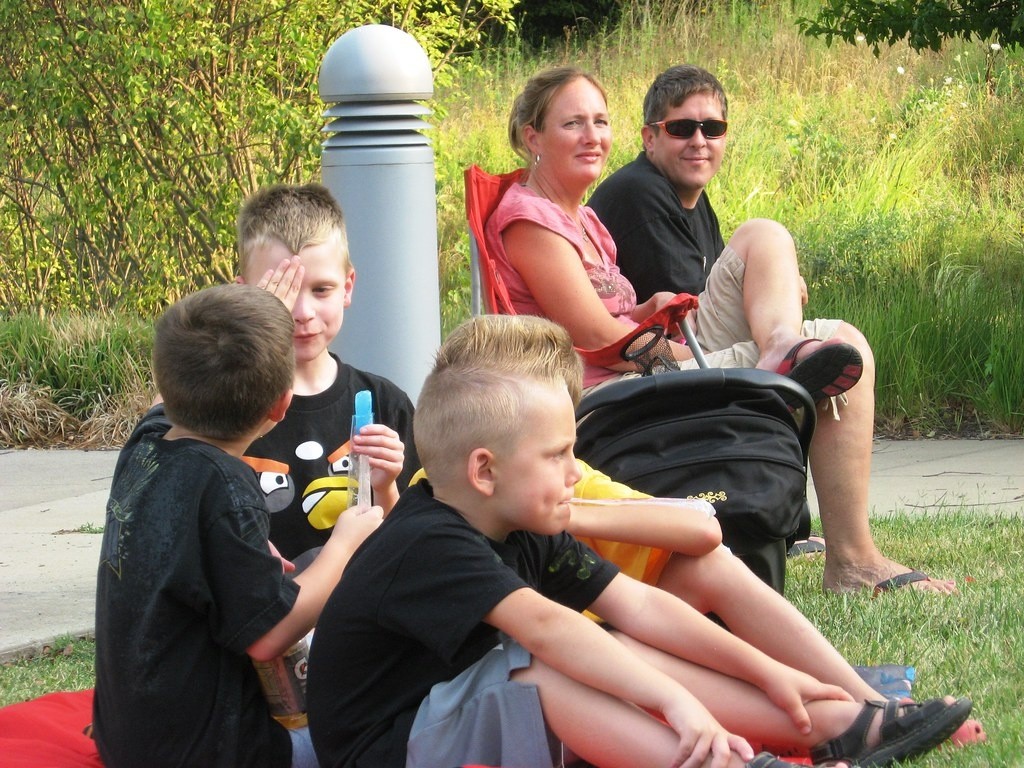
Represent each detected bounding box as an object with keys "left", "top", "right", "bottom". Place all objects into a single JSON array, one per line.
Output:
[
  {"left": 483, "top": 68, "right": 957, "bottom": 597},
  {"left": 586, "top": 65, "right": 826, "bottom": 562},
  {"left": 91, "top": 254, "right": 384, "bottom": 768},
  {"left": 304, "top": 316, "right": 974, "bottom": 768},
  {"left": 407, "top": 314, "right": 987, "bottom": 748},
  {"left": 234, "top": 181, "right": 423, "bottom": 581}
]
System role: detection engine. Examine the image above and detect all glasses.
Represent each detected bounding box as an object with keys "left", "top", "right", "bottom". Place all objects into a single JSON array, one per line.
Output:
[{"left": 649, "top": 118, "right": 729, "bottom": 138}]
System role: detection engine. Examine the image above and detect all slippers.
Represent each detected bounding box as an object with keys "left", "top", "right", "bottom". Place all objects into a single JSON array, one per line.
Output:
[
  {"left": 775, "top": 338, "right": 863, "bottom": 415},
  {"left": 871, "top": 566, "right": 959, "bottom": 603}
]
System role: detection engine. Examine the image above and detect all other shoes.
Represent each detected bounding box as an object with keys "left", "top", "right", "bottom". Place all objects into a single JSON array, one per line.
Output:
[{"left": 935, "top": 720, "right": 987, "bottom": 751}]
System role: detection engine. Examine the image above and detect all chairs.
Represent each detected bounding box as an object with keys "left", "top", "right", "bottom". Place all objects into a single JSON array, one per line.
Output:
[{"left": 465, "top": 165, "right": 824, "bottom": 559}]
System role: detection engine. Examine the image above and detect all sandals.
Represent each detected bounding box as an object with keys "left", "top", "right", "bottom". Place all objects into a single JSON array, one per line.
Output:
[
  {"left": 745, "top": 751, "right": 852, "bottom": 768},
  {"left": 810, "top": 695, "right": 972, "bottom": 768}
]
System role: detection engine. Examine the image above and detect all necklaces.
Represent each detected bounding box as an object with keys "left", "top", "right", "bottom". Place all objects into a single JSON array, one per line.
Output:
[{"left": 582, "top": 227, "right": 587, "bottom": 240}]
